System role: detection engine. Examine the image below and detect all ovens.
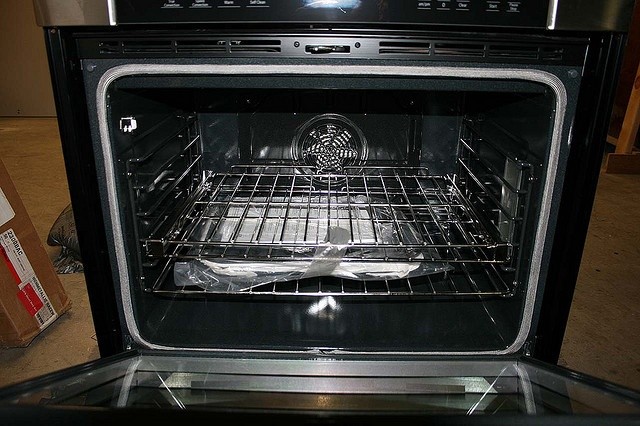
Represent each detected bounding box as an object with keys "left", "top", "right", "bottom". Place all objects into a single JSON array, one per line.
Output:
[{"left": 1, "top": 1, "right": 640, "bottom": 426}]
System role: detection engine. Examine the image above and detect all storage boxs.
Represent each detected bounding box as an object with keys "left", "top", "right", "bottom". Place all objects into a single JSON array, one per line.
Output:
[{"left": 1, "top": 158, "right": 72, "bottom": 348}]
